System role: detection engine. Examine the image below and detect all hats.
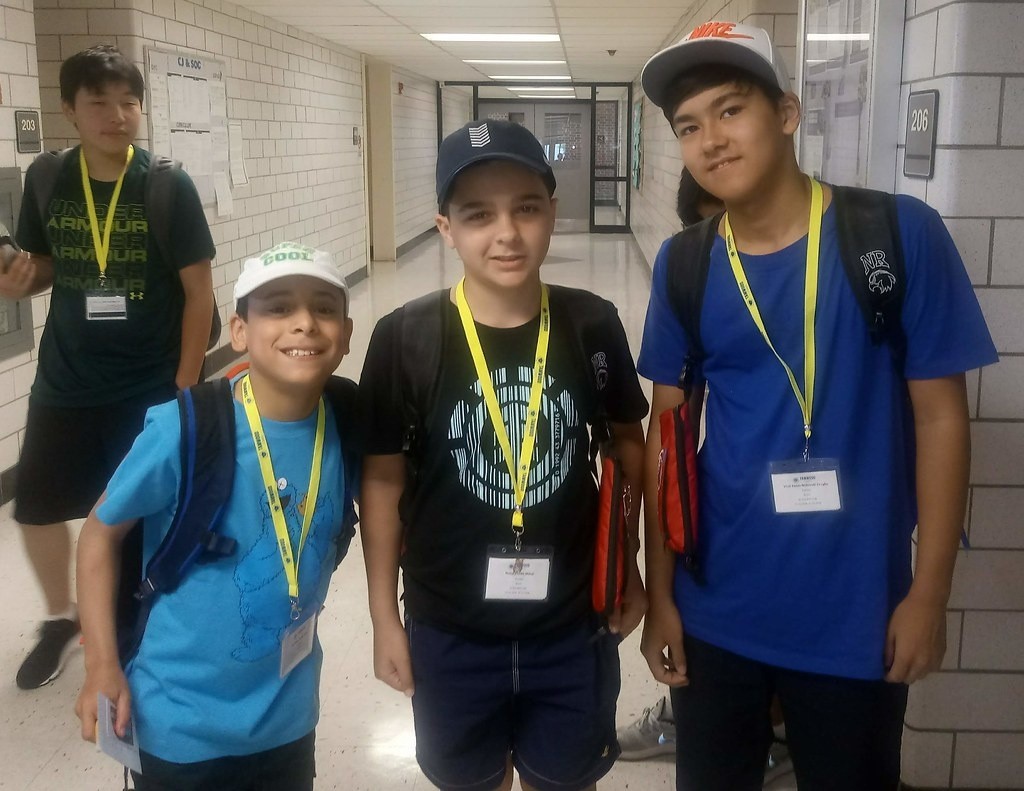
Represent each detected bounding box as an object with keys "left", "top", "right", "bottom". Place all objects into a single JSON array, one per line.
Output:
[
  {"left": 436, "top": 118, "right": 556, "bottom": 213},
  {"left": 641, "top": 21, "right": 793, "bottom": 108},
  {"left": 234, "top": 242, "right": 350, "bottom": 322}
]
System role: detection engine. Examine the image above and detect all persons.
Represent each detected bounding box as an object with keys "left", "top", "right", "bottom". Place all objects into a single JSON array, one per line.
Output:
[
  {"left": 617, "top": 17, "right": 999, "bottom": 791},
  {"left": 74, "top": 241, "right": 367, "bottom": 791},
  {"left": 357, "top": 121, "right": 649, "bottom": 791},
  {"left": 0, "top": 45, "right": 222, "bottom": 691}
]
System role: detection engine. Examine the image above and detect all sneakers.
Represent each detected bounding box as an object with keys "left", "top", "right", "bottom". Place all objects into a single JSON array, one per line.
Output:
[
  {"left": 17, "top": 612, "right": 81, "bottom": 689},
  {"left": 762, "top": 736, "right": 794, "bottom": 785},
  {"left": 611, "top": 697, "right": 677, "bottom": 759}
]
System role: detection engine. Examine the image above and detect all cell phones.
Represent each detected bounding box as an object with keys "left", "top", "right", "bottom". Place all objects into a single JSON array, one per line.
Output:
[{"left": 0, "top": 222, "right": 27, "bottom": 259}]
